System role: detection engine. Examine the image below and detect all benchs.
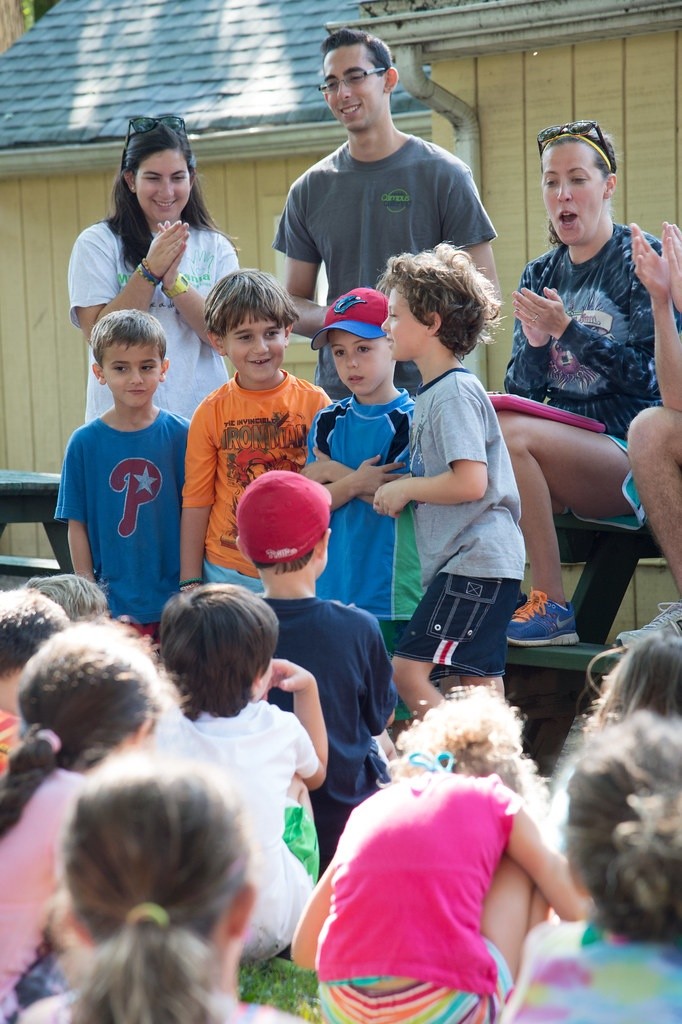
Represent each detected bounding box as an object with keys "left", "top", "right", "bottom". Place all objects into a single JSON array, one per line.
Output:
[{"left": 0, "top": 467, "right": 665, "bottom": 776}]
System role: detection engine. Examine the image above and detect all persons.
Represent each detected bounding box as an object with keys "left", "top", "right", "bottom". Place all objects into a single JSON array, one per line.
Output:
[
  {"left": 292, "top": 686, "right": 578, "bottom": 1024},
  {"left": 298, "top": 289, "right": 427, "bottom": 731},
  {"left": 274, "top": 29, "right": 498, "bottom": 398},
  {"left": 54, "top": 311, "right": 188, "bottom": 640},
  {"left": 235, "top": 470, "right": 400, "bottom": 872},
  {"left": 615, "top": 223, "right": 682, "bottom": 646},
  {"left": 499, "top": 642, "right": 682, "bottom": 1024},
  {"left": 0, "top": 576, "right": 327, "bottom": 1023},
  {"left": 375, "top": 246, "right": 527, "bottom": 716},
  {"left": 492, "top": 121, "right": 682, "bottom": 644},
  {"left": 178, "top": 268, "right": 335, "bottom": 598},
  {"left": 68, "top": 115, "right": 241, "bottom": 421}
]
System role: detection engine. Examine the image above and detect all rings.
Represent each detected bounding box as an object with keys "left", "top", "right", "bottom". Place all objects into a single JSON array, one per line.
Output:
[{"left": 533, "top": 316, "right": 538, "bottom": 321}]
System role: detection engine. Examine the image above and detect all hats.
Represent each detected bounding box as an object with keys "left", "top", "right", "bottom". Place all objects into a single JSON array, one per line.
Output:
[
  {"left": 310, "top": 287, "right": 389, "bottom": 351},
  {"left": 234, "top": 471, "right": 332, "bottom": 562}
]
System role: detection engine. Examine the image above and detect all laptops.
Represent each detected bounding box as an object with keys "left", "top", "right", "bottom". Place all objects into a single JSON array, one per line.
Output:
[{"left": 485, "top": 394, "right": 606, "bottom": 433}]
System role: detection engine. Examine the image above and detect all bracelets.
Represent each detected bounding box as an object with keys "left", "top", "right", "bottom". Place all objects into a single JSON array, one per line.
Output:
[
  {"left": 179, "top": 577, "right": 204, "bottom": 585},
  {"left": 135, "top": 258, "right": 162, "bottom": 286},
  {"left": 180, "top": 582, "right": 201, "bottom": 592}
]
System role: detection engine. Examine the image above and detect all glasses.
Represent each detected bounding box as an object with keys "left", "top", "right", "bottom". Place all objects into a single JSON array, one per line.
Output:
[
  {"left": 317, "top": 67, "right": 386, "bottom": 95},
  {"left": 537, "top": 120, "right": 613, "bottom": 170},
  {"left": 123, "top": 116, "right": 188, "bottom": 152}
]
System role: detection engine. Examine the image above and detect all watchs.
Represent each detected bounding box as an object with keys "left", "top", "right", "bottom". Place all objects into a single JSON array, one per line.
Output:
[{"left": 162, "top": 274, "right": 189, "bottom": 297}]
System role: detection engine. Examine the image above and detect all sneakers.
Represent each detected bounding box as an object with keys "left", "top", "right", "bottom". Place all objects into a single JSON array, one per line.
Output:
[{"left": 506, "top": 584, "right": 579, "bottom": 646}]
